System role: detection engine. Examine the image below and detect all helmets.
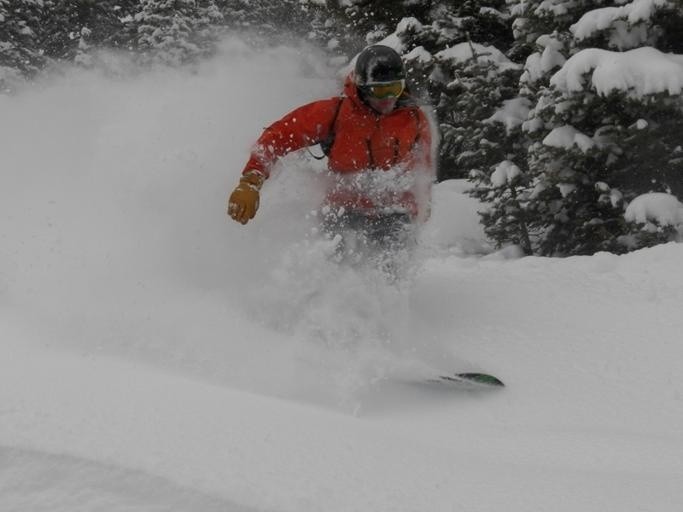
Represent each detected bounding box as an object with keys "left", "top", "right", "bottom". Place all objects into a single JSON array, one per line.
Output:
[{"left": 353, "top": 46, "right": 409, "bottom": 94}]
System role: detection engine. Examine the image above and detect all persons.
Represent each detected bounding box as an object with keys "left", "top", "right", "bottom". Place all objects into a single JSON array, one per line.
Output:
[{"left": 227, "top": 44, "right": 431, "bottom": 277}]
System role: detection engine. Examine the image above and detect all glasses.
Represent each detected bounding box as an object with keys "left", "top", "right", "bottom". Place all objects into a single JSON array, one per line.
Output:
[{"left": 352, "top": 68, "right": 406, "bottom": 101}]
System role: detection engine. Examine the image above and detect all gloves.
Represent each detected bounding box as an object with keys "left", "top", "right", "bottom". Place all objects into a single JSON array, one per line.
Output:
[{"left": 226, "top": 170, "right": 265, "bottom": 225}]
